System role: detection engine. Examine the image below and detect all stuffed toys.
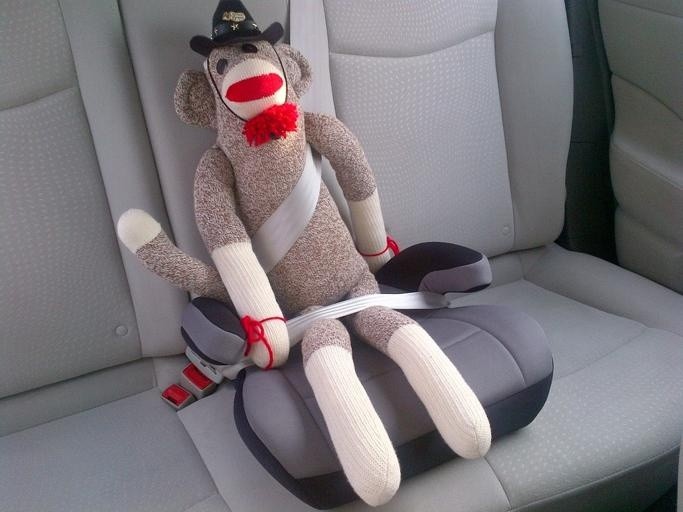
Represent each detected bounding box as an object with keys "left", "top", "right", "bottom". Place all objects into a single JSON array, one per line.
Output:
[{"left": 113, "top": 1, "right": 493, "bottom": 507}]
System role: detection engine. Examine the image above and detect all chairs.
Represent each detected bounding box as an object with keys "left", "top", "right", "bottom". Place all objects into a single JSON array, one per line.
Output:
[{"left": 1, "top": 1, "right": 682, "bottom": 510}]
